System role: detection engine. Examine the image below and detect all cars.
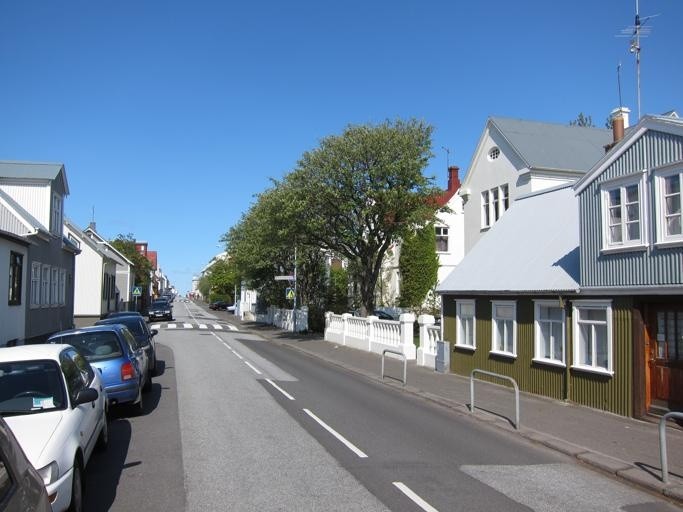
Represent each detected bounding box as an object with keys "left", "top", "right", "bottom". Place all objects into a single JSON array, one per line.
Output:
[
  {"left": 0, "top": 415, "right": 54, "bottom": 512},
  {"left": 46, "top": 324, "right": 153, "bottom": 414},
  {"left": 106, "top": 312, "right": 141, "bottom": 319},
  {"left": 350, "top": 310, "right": 398, "bottom": 321},
  {"left": 0, "top": 343, "right": 110, "bottom": 512},
  {"left": 93, "top": 317, "right": 157, "bottom": 374},
  {"left": 209, "top": 301, "right": 232, "bottom": 311},
  {"left": 227, "top": 303, "right": 237, "bottom": 314},
  {"left": 148, "top": 290, "right": 176, "bottom": 321}
]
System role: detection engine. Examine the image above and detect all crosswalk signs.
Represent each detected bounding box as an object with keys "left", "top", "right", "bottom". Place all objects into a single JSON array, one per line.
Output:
[
  {"left": 131, "top": 287, "right": 142, "bottom": 296},
  {"left": 286, "top": 288, "right": 295, "bottom": 299}
]
